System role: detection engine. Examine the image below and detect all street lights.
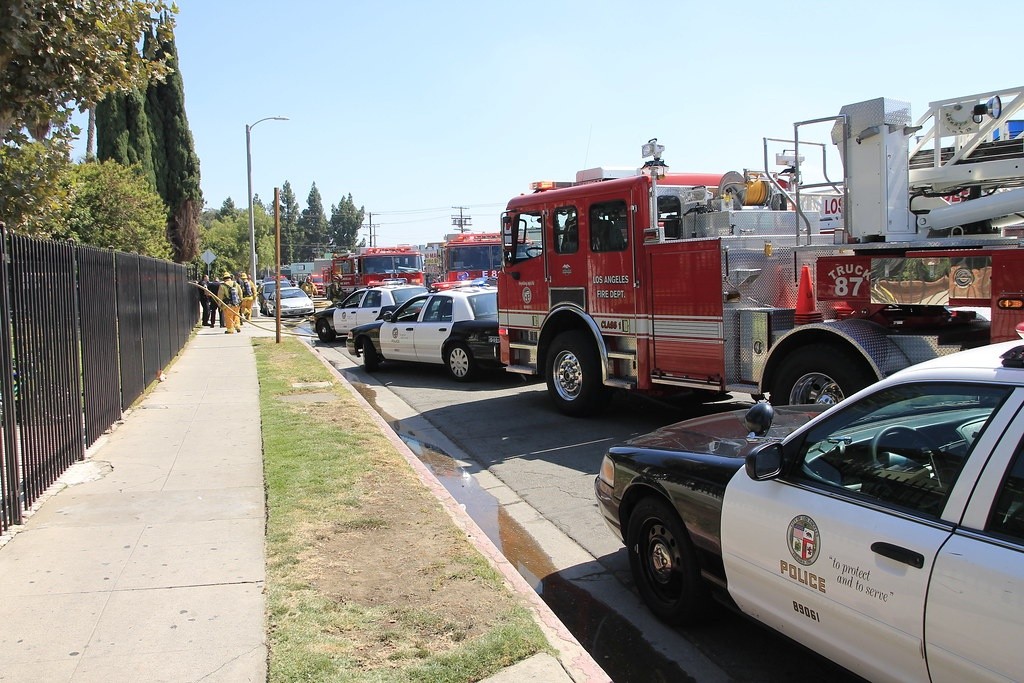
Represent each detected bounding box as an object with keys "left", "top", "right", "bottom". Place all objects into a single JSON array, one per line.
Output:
[{"left": 244, "top": 115, "right": 290, "bottom": 318}]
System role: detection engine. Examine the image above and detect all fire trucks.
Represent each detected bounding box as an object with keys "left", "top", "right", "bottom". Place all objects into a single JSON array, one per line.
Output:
[
  {"left": 332, "top": 246, "right": 424, "bottom": 298},
  {"left": 497, "top": 86, "right": 1024, "bottom": 417},
  {"left": 442, "top": 233, "right": 534, "bottom": 282}
]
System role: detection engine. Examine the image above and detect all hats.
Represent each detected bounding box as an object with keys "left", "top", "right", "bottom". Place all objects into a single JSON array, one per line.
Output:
[
  {"left": 241, "top": 273, "right": 248, "bottom": 279},
  {"left": 223, "top": 272, "right": 231, "bottom": 279},
  {"left": 306, "top": 276, "right": 311, "bottom": 280}
]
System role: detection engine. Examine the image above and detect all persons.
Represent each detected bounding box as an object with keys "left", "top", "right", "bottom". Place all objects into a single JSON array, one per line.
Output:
[
  {"left": 299, "top": 276, "right": 318, "bottom": 299},
  {"left": 290, "top": 277, "right": 304, "bottom": 287},
  {"left": 198, "top": 272, "right": 258, "bottom": 335},
  {"left": 328, "top": 274, "right": 343, "bottom": 304}
]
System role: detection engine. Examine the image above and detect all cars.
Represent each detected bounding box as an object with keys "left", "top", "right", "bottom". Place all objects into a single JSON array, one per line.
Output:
[
  {"left": 346, "top": 282, "right": 500, "bottom": 380},
  {"left": 594, "top": 321, "right": 1024, "bottom": 682},
  {"left": 309, "top": 281, "right": 428, "bottom": 343},
  {"left": 257, "top": 282, "right": 293, "bottom": 312},
  {"left": 266, "top": 287, "right": 316, "bottom": 317}
]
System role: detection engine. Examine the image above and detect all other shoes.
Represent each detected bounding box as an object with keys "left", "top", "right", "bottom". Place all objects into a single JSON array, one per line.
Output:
[
  {"left": 202, "top": 324, "right": 211, "bottom": 326},
  {"left": 237, "top": 330, "right": 240, "bottom": 332},
  {"left": 225, "top": 331, "right": 233, "bottom": 333}
]
